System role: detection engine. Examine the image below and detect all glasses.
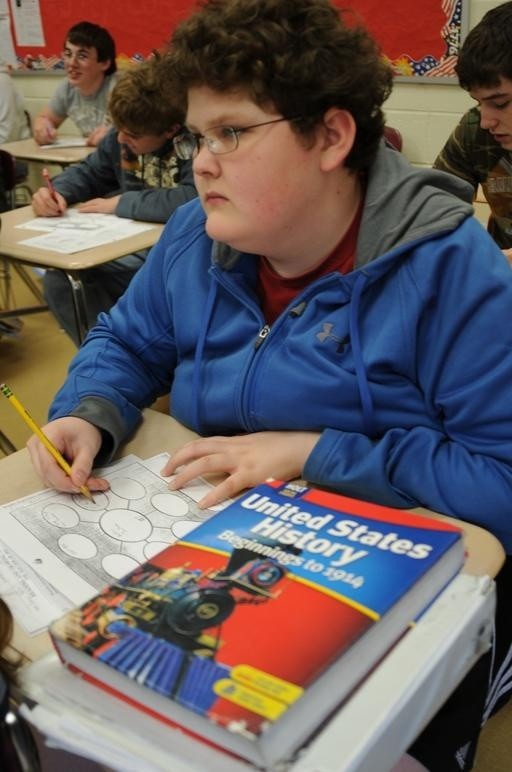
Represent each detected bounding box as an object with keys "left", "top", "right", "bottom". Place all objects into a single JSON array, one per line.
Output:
[
  {"left": 61, "top": 50, "right": 102, "bottom": 60},
  {"left": 172, "top": 115, "right": 300, "bottom": 161}
]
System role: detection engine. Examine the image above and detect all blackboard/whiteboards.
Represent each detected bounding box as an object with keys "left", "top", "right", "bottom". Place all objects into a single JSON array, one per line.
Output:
[{"left": 1, "top": 1, "right": 468, "bottom": 77}]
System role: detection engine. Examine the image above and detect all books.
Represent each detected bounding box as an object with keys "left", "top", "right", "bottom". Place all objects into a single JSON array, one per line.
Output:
[{"left": 47, "top": 477, "right": 465, "bottom": 772}]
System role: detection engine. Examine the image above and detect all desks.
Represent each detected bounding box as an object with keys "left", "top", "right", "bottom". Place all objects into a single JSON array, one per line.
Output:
[
  {"left": 0, "top": 202, "right": 166, "bottom": 348},
  {"left": 0, "top": 407, "right": 506, "bottom": 772},
  {"left": 0, "top": 134, "right": 98, "bottom": 209}
]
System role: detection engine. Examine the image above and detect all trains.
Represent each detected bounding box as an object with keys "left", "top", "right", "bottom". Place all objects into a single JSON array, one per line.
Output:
[{"left": 78, "top": 538, "right": 307, "bottom": 720}]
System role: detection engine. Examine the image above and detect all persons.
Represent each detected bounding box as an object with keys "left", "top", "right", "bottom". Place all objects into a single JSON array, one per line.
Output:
[
  {"left": 0, "top": 64, "right": 29, "bottom": 191},
  {"left": 31, "top": 22, "right": 123, "bottom": 147},
  {"left": 27, "top": 1, "right": 512, "bottom": 772},
  {"left": 32, "top": 63, "right": 199, "bottom": 224},
  {"left": 432, "top": 1, "right": 512, "bottom": 263}
]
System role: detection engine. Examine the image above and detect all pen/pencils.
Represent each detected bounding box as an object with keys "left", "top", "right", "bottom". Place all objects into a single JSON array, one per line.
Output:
[
  {"left": 1, "top": 384, "right": 96, "bottom": 504},
  {"left": 41, "top": 168, "right": 62, "bottom": 216}
]
